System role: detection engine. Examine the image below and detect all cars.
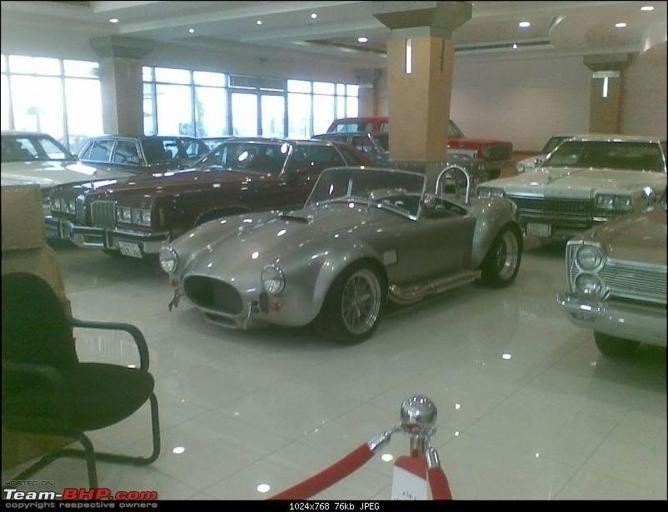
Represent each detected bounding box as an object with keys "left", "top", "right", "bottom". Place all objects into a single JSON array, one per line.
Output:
[
  {"left": 312, "top": 129, "right": 474, "bottom": 188},
  {"left": 78, "top": 133, "right": 212, "bottom": 172},
  {"left": 556, "top": 184, "right": 668, "bottom": 354},
  {"left": 65, "top": 136, "right": 386, "bottom": 260},
  {"left": 1, "top": 130, "right": 137, "bottom": 243},
  {"left": 478, "top": 132, "right": 667, "bottom": 243}
]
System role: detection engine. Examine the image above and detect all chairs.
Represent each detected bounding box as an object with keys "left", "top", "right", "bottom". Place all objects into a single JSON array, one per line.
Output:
[{"left": 1, "top": 272, "right": 161, "bottom": 494}]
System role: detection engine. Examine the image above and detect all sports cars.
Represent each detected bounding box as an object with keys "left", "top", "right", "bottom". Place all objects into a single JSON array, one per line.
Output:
[{"left": 156, "top": 163, "right": 522, "bottom": 344}]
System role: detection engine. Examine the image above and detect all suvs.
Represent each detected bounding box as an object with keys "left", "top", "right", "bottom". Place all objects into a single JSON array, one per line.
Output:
[{"left": 325, "top": 118, "right": 513, "bottom": 179}]
